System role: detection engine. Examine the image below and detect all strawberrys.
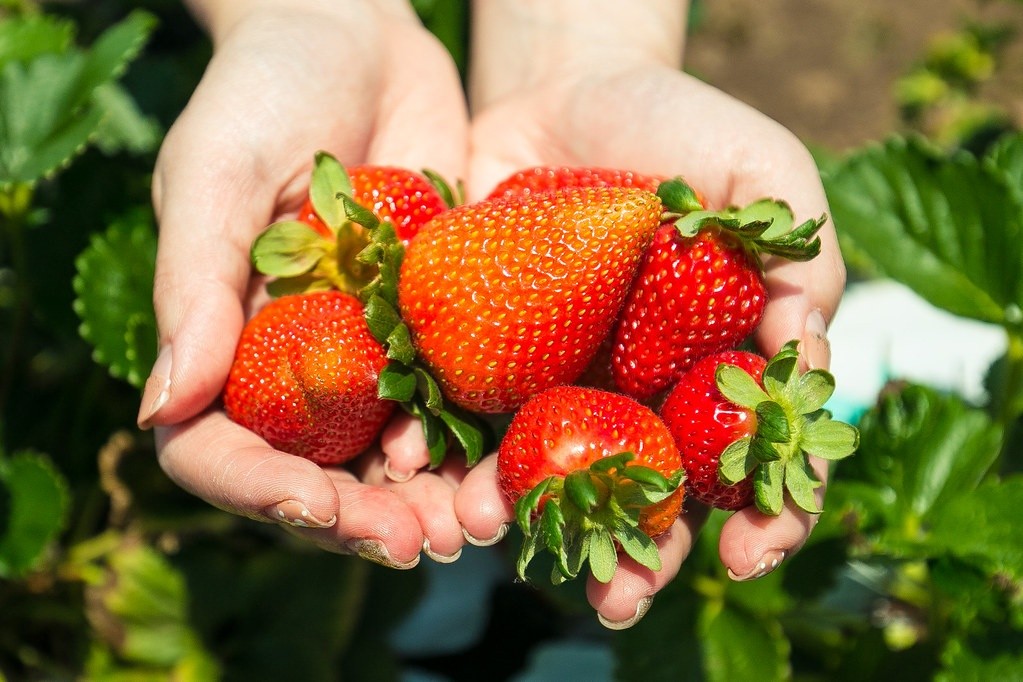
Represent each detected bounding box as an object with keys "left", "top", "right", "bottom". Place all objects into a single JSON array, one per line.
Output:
[{"left": 224, "top": 165, "right": 776, "bottom": 548}]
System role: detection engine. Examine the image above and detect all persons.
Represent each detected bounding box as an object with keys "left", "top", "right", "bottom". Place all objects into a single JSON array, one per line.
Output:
[{"left": 137, "top": 0, "right": 848, "bottom": 630}]
